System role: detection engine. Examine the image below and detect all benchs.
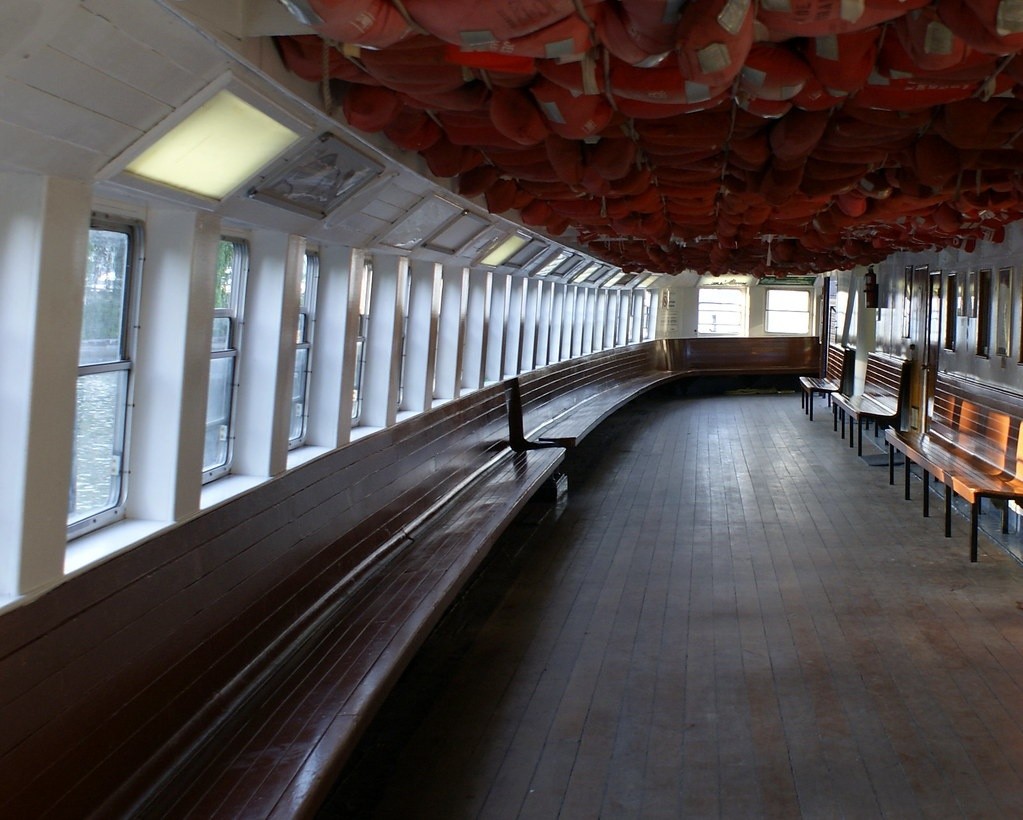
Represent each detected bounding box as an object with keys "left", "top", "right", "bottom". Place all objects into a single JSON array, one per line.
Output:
[
  {"left": 511, "top": 339, "right": 693, "bottom": 451},
  {"left": 885, "top": 370, "right": 1023, "bottom": 564},
  {"left": 0, "top": 380, "right": 570, "bottom": 820},
  {"left": 830, "top": 351, "right": 911, "bottom": 457},
  {"left": 663, "top": 336, "right": 821, "bottom": 377},
  {"left": 799, "top": 344, "right": 851, "bottom": 421}
]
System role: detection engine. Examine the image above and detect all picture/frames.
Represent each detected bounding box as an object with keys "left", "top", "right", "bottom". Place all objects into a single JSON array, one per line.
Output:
[
  {"left": 956, "top": 273, "right": 966, "bottom": 316},
  {"left": 966, "top": 270, "right": 977, "bottom": 319},
  {"left": 900, "top": 265, "right": 913, "bottom": 338},
  {"left": 996, "top": 266, "right": 1012, "bottom": 358}
]
